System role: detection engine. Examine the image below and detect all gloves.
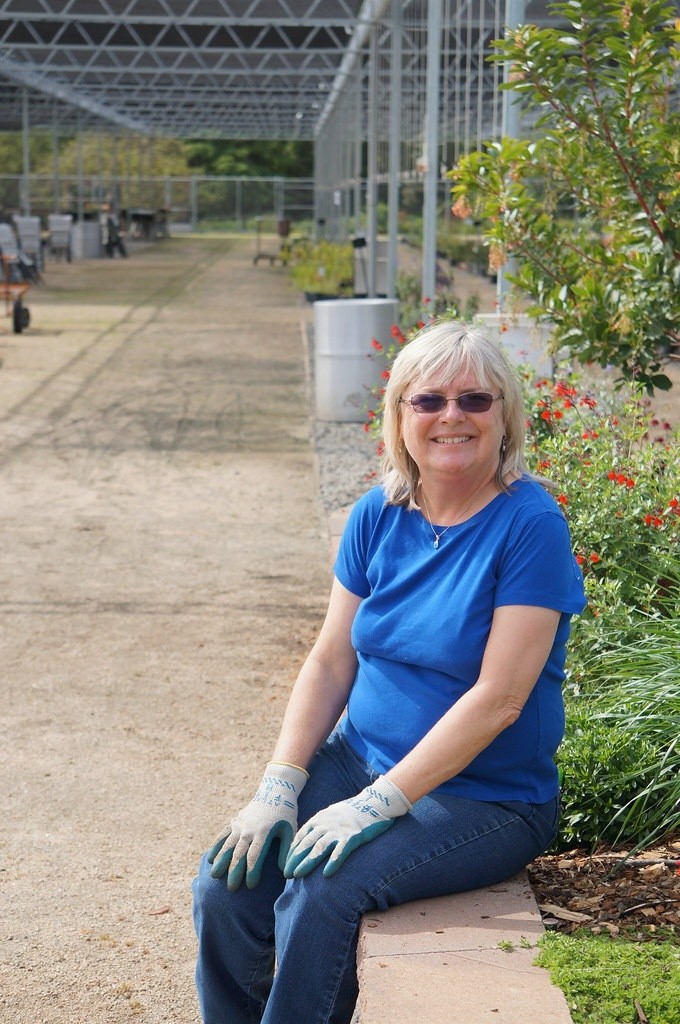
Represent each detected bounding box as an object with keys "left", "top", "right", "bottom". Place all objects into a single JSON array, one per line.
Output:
[
  {"left": 207, "top": 761, "right": 309, "bottom": 891},
  {"left": 284, "top": 774, "right": 412, "bottom": 879}
]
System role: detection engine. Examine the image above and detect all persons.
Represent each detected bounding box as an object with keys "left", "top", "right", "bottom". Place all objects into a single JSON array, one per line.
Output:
[{"left": 183, "top": 315, "right": 591, "bottom": 1024}]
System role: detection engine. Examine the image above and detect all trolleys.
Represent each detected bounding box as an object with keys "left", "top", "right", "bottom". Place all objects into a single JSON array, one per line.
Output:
[{"left": 0, "top": 256, "right": 30, "bottom": 334}]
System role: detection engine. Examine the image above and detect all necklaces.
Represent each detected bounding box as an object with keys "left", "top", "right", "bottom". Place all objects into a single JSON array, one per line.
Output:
[{"left": 416, "top": 465, "right": 497, "bottom": 550}]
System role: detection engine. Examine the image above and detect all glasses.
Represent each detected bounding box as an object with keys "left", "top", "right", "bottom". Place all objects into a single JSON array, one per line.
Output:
[{"left": 399, "top": 393, "right": 504, "bottom": 413}]
